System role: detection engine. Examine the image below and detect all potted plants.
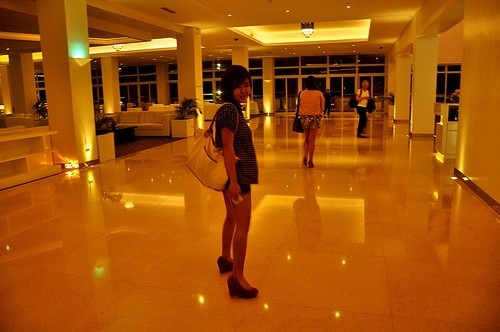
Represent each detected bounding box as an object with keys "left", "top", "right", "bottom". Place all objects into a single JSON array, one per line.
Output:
[
  {"left": 171, "top": 98, "right": 203, "bottom": 139},
  {"left": 94, "top": 114, "right": 119, "bottom": 163},
  {"left": 386, "top": 92, "right": 394, "bottom": 119},
  {"left": 32, "top": 100, "right": 49, "bottom": 126}
]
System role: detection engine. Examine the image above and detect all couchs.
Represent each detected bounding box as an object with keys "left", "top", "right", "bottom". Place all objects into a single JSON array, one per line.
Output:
[
  {"left": 110, "top": 101, "right": 230, "bottom": 138},
  {"left": 0, "top": 114, "right": 34, "bottom": 132}
]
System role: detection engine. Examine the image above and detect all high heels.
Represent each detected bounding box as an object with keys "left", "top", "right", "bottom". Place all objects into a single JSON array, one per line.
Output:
[
  {"left": 308, "top": 161, "right": 314, "bottom": 168},
  {"left": 217, "top": 256, "right": 233, "bottom": 274},
  {"left": 227, "top": 276, "right": 259, "bottom": 297},
  {"left": 302, "top": 157, "right": 308, "bottom": 166}
]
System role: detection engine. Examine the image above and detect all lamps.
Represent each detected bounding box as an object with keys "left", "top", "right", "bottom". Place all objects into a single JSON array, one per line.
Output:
[
  {"left": 300, "top": 22, "right": 315, "bottom": 38},
  {"left": 75, "top": 57, "right": 93, "bottom": 67},
  {"left": 113, "top": 44, "right": 123, "bottom": 51}
]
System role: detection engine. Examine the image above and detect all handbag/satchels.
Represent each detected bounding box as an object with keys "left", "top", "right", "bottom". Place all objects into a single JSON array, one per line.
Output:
[{"left": 293, "top": 118, "right": 304, "bottom": 134}]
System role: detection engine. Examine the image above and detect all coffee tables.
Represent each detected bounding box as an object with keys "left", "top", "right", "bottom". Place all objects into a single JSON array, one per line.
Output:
[{"left": 97, "top": 125, "right": 138, "bottom": 143}]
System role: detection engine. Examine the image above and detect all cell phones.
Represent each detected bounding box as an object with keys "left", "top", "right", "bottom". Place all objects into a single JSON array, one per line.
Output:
[{"left": 232, "top": 194, "right": 243, "bottom": 205}]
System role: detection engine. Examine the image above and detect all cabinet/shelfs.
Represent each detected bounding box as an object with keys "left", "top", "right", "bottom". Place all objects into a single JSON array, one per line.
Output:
[
  {"left": 0, "top": 125, "right": 62, "bottom": 189},
  {"left": 434, "top": 103, "right": 459, "bottom": 162}
]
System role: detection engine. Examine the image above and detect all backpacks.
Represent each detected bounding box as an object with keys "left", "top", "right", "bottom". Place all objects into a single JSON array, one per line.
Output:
[
  {"left": 184, "top": 103, "right": 239, "bottom": 191},
  {"left": 348, "top": 88, "right": 362, "bottom": 108}
]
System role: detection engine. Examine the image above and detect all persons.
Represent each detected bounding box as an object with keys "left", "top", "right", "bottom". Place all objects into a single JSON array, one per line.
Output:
[
  {"left": 324, "top": 89, "right": 332, "bottom": 115},
  {"left": 355, "top": 79, "right": 372, "bottom": 138},
  {"left": 295, "top": 75, "right": 325, "bottom": 168},
  {"left": 213, "top": 65, "right": 259, "bottom": 298}
]
